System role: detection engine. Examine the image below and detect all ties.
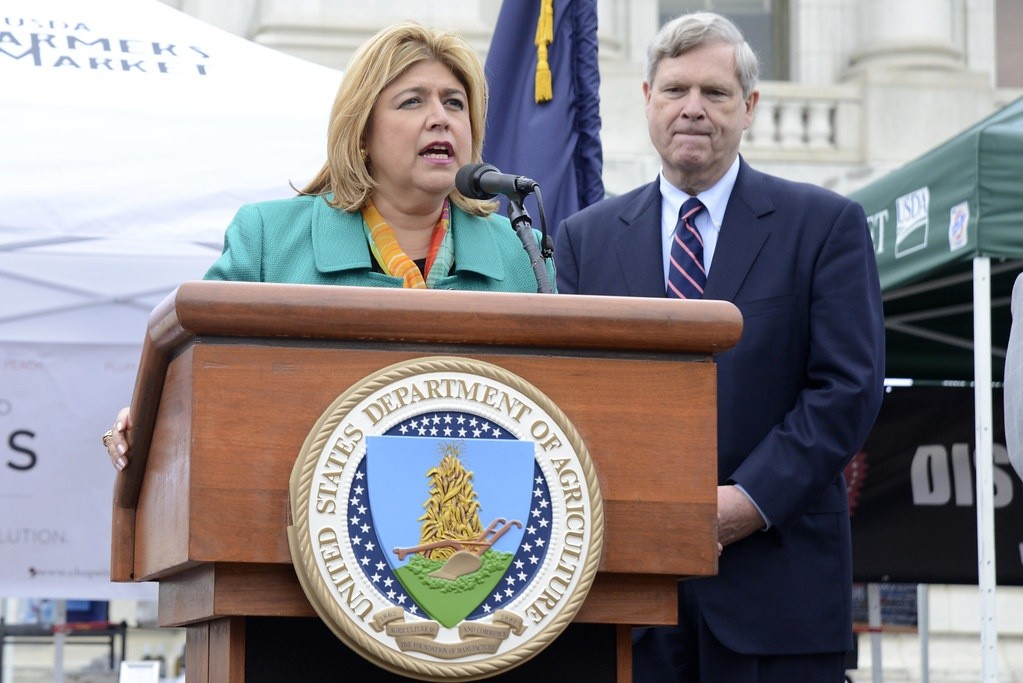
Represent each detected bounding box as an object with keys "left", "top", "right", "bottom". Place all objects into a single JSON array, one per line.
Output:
[{"left": 666, "top": 198, "right": 705, "bottom": 300}]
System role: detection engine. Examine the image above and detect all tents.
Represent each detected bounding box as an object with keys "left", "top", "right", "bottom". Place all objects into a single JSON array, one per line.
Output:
[
  {"left": 845, "top": 91, "right": 1022, "bottom": 680},
  {"left": 0, "top": 4, "right": 348, "bottom": 596}
]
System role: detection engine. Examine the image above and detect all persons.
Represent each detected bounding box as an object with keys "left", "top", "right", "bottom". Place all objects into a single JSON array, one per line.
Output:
[
  {"left": 103, "top": 22, "right": 560, "bottom": 476},
  {"left": 553, "top": 7, "right": 883, "bottom": 683}
]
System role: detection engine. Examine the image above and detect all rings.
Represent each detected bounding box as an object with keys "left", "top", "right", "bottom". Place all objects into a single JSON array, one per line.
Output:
[{"left": 102, "top": 432, "right": 112, "bottom": 446}]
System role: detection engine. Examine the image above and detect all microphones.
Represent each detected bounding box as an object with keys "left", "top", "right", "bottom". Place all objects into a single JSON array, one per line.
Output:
[{"left": 454, "top": 163, "right": 539, "bottom": 200}]
